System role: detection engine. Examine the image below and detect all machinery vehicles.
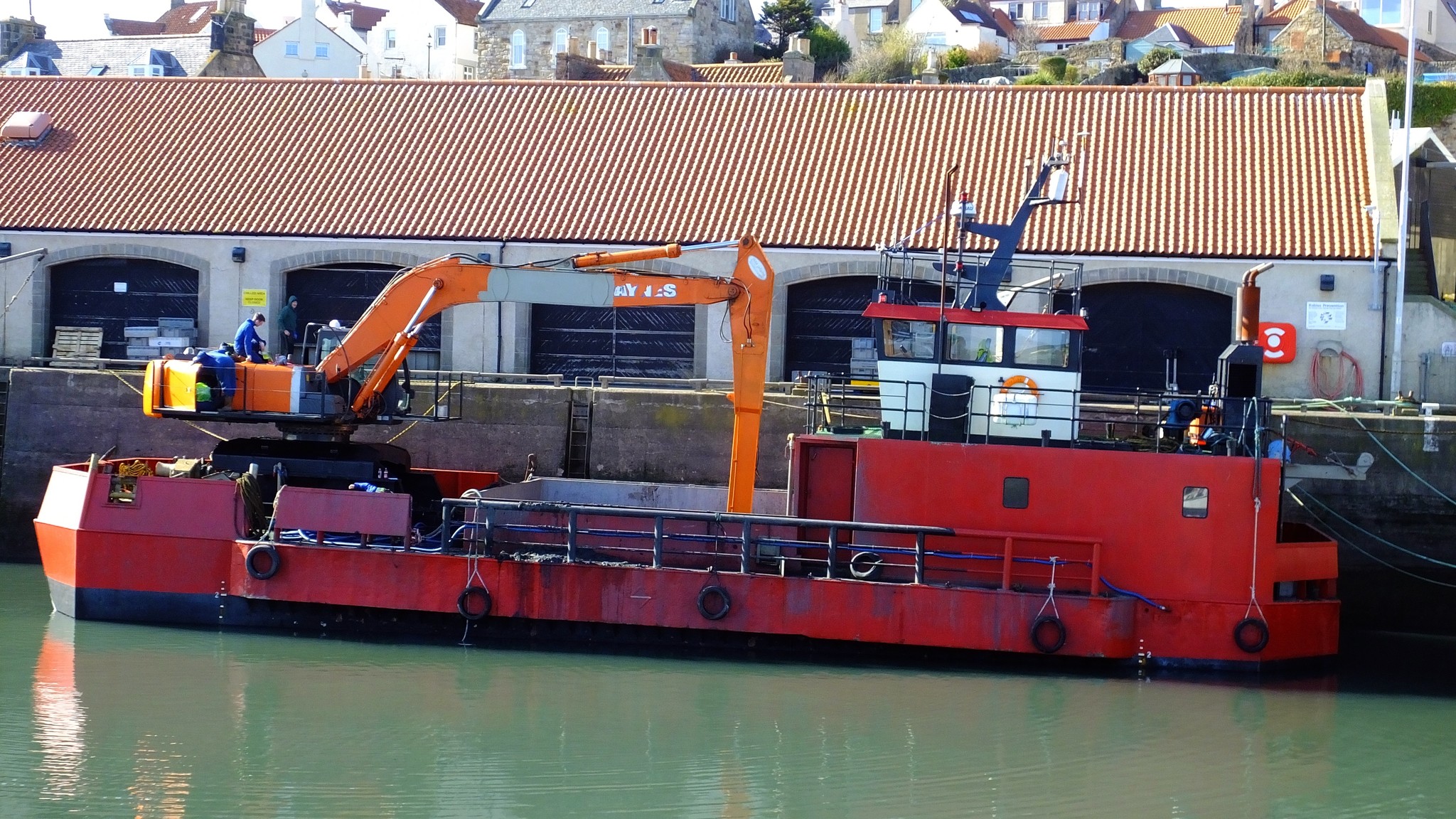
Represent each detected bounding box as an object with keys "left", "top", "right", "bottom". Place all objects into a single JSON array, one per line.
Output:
[{"left": 144, "top": 231, "right": 777, "bottom": 513}]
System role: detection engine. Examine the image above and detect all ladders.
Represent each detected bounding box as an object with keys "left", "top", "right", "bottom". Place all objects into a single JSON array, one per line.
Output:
[{"left": 567, "top": 401, "right": 590, "bottom": 479}]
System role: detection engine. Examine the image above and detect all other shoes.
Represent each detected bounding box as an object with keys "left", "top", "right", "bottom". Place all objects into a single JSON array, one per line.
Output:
[
  {"left": 232, "top": 354, "right": 246, "bottom": 362},
  {"left": 219, "top": 396, "right": 233, "bottom": 411}
]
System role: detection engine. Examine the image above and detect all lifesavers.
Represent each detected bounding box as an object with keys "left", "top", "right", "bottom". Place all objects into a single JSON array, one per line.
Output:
[
  {"left": 1001, "top": 375, "right": 1039, "bottom": 402},
  {"left": 1176, "top": 400, "right": 1196, "bottom": 421},
  {"left": 696, "top": 584, "right": 732, "bottom": 621},
  {"left": 1232, "top": 617, "right": 1269, "bottom": 654},
  {"left": 246, "top": 544, "right": 280, "bottom": 581},
  {"left": 457, "top": 586, "right": 491, "bottom": 618},
  {"left": 1029, "top": 614, "right": 1066, "bottom": 655}
]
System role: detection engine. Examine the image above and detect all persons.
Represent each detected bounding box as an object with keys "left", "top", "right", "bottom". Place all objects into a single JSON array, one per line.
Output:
[{"left": 191, "top": 296, "right": 299, "bottom": 413}]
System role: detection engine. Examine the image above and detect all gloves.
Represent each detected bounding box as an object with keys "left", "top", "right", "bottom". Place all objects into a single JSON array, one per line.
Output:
[
  {"left": 293, "top": 331, "right": 297, "bottom": 338},
  {"left": 260, "top": 342, "right": 265, "bottom": 351},
  {"left": 246, "top": 355, "right": 251, "bottom": 361}
]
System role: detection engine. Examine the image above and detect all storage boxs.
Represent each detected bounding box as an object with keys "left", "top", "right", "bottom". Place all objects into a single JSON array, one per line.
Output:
[{"left": 123, "top": 317, "right": 200, "bottom": 362}]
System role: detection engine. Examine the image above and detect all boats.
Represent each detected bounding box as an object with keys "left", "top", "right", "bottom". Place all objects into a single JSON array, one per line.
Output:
[{"left": 38, "top": 132, "right": 1456, "bottom": 694}]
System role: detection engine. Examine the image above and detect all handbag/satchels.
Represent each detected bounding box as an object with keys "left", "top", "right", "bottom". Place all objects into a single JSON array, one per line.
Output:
[{"left": 275, "top": 354, "right": 287, "bottom": 367}]
